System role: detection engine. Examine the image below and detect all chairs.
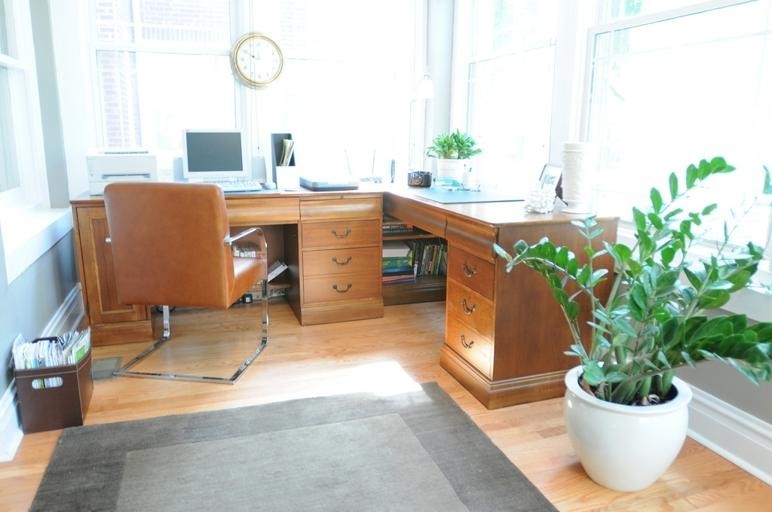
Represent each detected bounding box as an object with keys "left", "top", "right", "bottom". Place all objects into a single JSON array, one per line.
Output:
[{"left": 103, "top": 181, "right": 269, "bottom": 386}]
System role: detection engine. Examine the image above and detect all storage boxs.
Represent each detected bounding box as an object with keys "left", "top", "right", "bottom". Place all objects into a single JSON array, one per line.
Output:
[{"left": 13, "top": 349, "right": 94, "bottom": 437}]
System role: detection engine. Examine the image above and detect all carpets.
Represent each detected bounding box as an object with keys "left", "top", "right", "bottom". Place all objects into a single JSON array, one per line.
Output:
[{"left": 27, "top": 381, "right": 563, "bottom": 512}]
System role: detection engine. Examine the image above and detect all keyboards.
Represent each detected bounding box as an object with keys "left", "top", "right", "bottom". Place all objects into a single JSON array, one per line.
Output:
[{"left": 196, "top": 182, "right": 261, "bottom": 191}]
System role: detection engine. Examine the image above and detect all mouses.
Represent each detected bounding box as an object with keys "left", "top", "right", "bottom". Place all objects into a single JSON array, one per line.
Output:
[{"left": 264, "top": 182, "right": 276, "bottom": 189}]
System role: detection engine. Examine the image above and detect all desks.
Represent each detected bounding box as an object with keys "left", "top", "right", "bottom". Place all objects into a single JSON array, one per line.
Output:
[
  {"left": 383, "top": 180, "right": 621, "bottom": 412},
  {"left": 67, "top": 178, "right": 386, "bottom": 349}
]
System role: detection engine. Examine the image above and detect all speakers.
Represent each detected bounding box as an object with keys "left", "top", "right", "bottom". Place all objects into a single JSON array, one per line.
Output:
[
  {"left": 174, "top": 158, "right": 184, "bottom": 181},
  {"left": 252, "top": 157, "right": 265, "bottom": 180}
]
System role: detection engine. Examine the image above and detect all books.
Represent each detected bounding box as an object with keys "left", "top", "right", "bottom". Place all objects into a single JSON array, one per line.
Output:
[
  {"left": 10, "top": 324, "right": 91, "bottom": 370},
  {"left": 383, "top": 218, "right": 448, "bottom": 286}
]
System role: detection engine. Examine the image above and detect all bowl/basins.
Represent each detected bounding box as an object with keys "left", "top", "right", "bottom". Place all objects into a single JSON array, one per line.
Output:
[{"left": 407, "top": 171, "right": 432, "bottom": 188}]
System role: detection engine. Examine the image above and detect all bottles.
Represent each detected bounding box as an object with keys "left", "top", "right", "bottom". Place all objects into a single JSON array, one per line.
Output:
[{"left": 172, "top": 157, "right": 184, "bottom": 181}]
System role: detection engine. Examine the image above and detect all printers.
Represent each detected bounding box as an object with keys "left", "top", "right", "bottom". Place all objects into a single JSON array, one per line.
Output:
[{"left": 84, "top": 153, "right": 155, "bottom": 193}]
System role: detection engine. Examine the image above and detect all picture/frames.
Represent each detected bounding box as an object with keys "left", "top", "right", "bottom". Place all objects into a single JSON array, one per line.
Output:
[{"left": 538, "top": 162, "right": 568, "bottom": 207}]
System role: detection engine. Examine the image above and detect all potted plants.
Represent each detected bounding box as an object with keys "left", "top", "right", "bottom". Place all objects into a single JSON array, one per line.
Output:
[
  {"left": 491, "top": 152, "right": 772, "bottom": 495},
  {"left": 422, "top": 127, "right": 483, "bottom": 185}
]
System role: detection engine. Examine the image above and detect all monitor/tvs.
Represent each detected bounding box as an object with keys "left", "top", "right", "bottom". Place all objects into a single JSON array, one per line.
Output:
[{"left": 182, "top": 129, "right": 246, "bottom": 177}]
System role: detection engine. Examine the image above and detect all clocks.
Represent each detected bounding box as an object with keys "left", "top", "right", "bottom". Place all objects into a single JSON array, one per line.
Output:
[{"left": 231, "top": 31, "right": 285, "bottom": 89}]
